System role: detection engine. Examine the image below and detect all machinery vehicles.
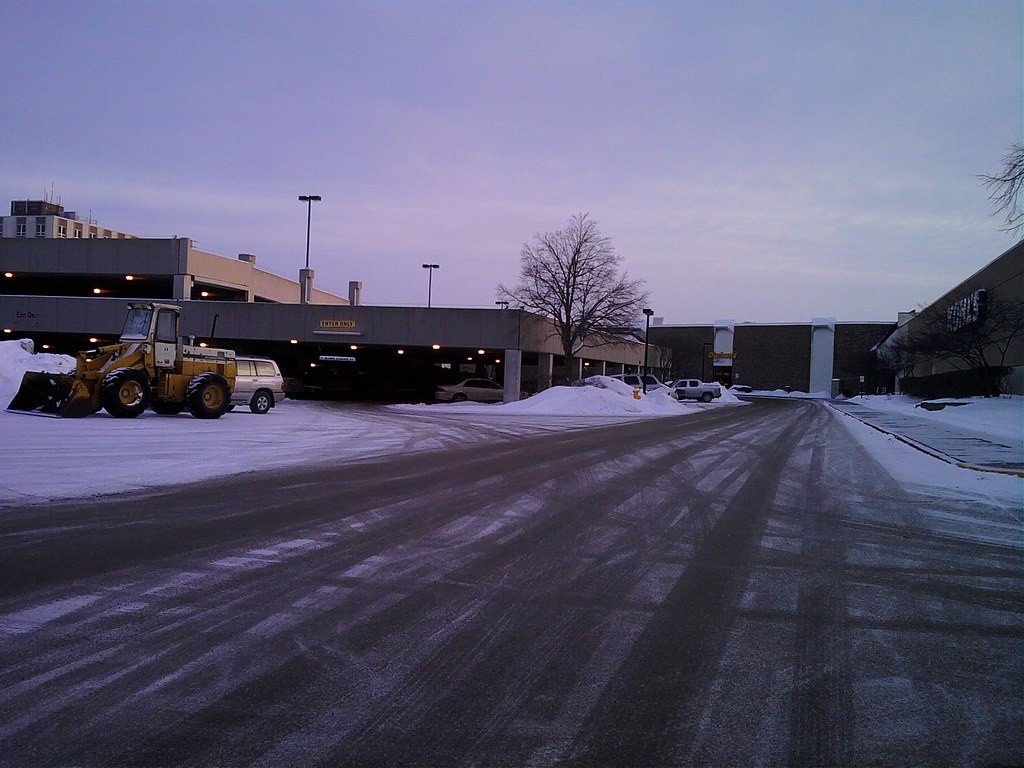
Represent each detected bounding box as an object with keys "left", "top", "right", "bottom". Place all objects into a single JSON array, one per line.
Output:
[{"left": 6, "top": 298, "right": 237, "bottom": 419}]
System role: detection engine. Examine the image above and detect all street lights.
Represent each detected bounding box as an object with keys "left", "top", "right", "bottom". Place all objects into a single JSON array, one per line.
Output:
[
  {"left": 422, "top": 263, "right": 440, "bottom": 308},
  {"left": 298, "top": 195, "right": 321, "bottom": 269},
  {"left": 642, "top": 308, "right": 654, "bottom": 395},
  {"left": 496, "top": 301, "right": 509, "bottom": 310}
]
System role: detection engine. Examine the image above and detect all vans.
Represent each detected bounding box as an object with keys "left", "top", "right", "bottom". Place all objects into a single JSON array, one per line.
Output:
[{"left": 608, "top": 372, "right": 678, "bottom": 399}]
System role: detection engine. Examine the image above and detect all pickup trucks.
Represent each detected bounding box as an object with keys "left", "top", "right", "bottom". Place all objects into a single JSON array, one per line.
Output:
[{"left": 669, "top": 378, "right": 722, "bottom": 403}]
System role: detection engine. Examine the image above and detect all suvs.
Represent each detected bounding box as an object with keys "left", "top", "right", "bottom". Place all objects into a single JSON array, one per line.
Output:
[{"left": 225, "top": 355, "right": 286, "bottom": 414}]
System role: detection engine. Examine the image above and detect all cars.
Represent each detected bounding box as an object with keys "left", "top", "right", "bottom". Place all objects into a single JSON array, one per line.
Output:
[{"left": 435, "top": 376, "right": 529, "bottom": 403}]
